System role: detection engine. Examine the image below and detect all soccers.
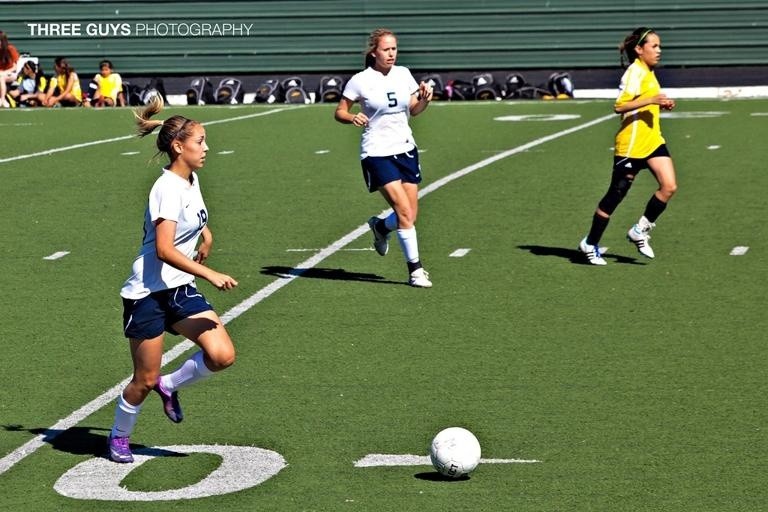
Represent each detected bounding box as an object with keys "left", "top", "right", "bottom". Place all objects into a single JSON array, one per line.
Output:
[{"left": 430, "top": 425, "right": 483, "bottom": 478}]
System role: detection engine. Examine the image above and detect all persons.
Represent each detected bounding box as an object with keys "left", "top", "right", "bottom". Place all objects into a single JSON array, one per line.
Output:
[
  {"left": 335, "top": 27, "right": 433, "bottom": 287},
  {"left": 8, "top": 61, "right": 47, "bottom": 107},
  {"left": 109, "top": 95, "right": 237, "bottom": 462},
  {"left": 0, "top": 32, "right": 18, "bottom": 106},
  {"left": 42, "top": 58, "right": 83, "bottom": 108},
  {"left": 86, "top": 60, "right": 126, "bottom": 109},
  {"left": 578, "top": 28, "right": 676, "bottom": 265}
]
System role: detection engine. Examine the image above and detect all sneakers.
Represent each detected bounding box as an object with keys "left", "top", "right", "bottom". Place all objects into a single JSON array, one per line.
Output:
[
  {"left": 370, "top": 216, "right": 388, "bottom": 257},
  {"left": 156, "top": 376, "right": 184, "bottom": 423},
  {"left": 0, "top": 93, "right": 20, "bottom": 108},
  {"left": 627, "top": 226, "right": 655, "bottom": 259},
  {"left": 408, "top": 268, "right": 432, "bottom": 287},
  {"left": 579, "top": 238, "right": 608, "bottom": 266},
  {"left": 108, "top": 433, "right": 134, "bottom": 464}
]
